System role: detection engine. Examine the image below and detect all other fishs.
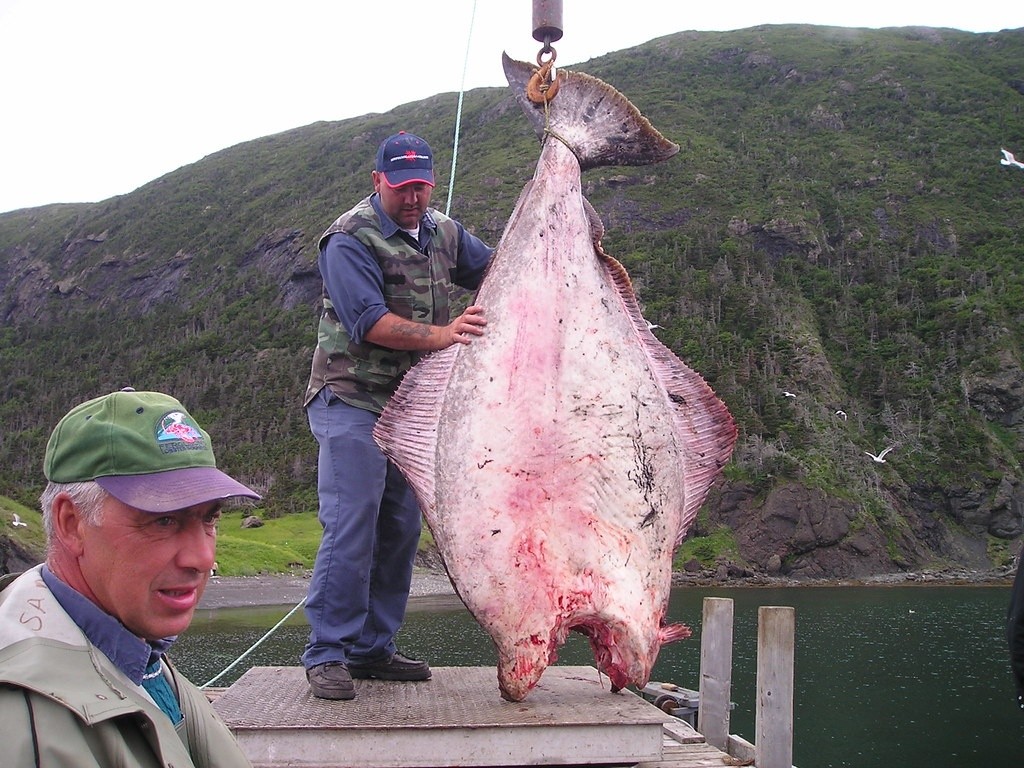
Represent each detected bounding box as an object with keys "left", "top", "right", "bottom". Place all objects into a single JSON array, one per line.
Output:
[{"left": 371, "top": 51, "right": 738, "bottom": 702}]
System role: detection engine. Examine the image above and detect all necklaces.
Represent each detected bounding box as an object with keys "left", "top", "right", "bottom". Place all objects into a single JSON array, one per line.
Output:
[{"left": 142, "top": 657, "right": 163, "bottom": 680}]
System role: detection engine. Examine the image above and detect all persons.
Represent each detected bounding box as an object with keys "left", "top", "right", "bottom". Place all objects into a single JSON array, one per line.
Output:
[
  {"left": 299, "top": 130, "right": 503, "bottom": 698},
  {"left": 0, "top": 384, "right": 262, "bottom": 768}
]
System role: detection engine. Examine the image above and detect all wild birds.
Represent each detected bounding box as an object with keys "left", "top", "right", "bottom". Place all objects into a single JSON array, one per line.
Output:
[
  {"left": 836, "top": 410, "right": 847, "bottom": 422},
  {"left": 861, "top": 446, "right": 893, "bottom": 463},
  {"left": 11, "top": 512, "right": 28, "bottom": 527},
  {"left": 641, "top": 319, "right": 666, "bottom": 330},
  {"left": 781, "top": 392, "right": 796, "bottom": 398},
  {"left": 999, "top": 150, "right": 1024, "bottom": 169}
]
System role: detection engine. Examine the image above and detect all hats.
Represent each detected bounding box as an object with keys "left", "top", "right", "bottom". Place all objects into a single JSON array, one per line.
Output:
[
  {"left": 375, "top": 131, "right": 436, "bottom": 190},
  {"left": 44, "top": 387, "right": 262, "bottom": 515}
]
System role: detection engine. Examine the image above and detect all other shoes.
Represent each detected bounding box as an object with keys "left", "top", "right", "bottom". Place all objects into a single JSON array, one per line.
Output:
[
  {"left": 306, "top": 662, "right": 357, "bottom": 702},
  {"left": 348, "top": 648, "right": 431, "bottom": 681}
]
